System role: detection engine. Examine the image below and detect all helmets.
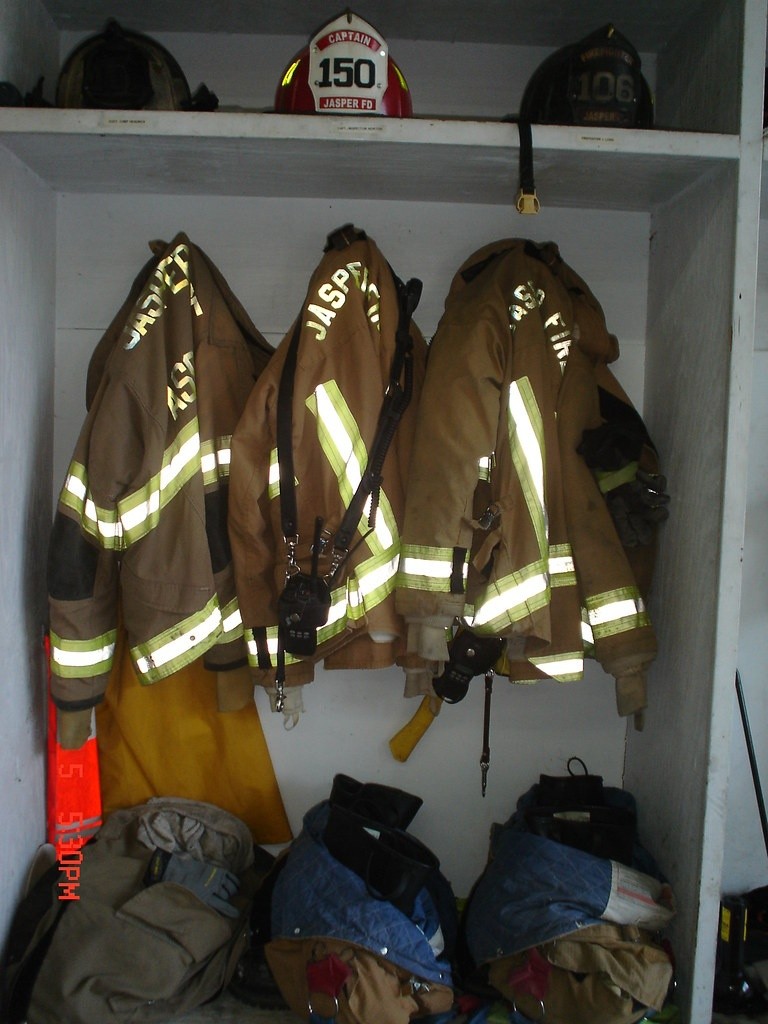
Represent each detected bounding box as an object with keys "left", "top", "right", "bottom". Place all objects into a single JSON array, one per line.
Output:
[
  {"left": 275, "top": 8, "right": 413, "bottom": 118},
  {"left": 53, "top": 19, "right": 219, "bottom": 112}
]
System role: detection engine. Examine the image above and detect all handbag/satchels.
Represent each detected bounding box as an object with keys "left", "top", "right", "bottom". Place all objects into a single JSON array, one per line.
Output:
[{"left": 0, "top": 795, "right": 255, "bottom": 1024}]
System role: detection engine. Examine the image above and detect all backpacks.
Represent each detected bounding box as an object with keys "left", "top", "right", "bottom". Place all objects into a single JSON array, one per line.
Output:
[{"left": 503, "top": 753, "right": 654, "bottom": 879}]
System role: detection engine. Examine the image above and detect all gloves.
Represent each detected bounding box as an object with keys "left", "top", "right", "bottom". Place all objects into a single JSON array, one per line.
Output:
[
  {"left": 577, "top": 423, "right": 670, "bottom": 548},
  {"left": 144, "top": 847, "right": 243, "bottom": 919}
]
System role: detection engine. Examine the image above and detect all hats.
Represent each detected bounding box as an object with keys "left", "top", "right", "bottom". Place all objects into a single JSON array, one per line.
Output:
[{"left": 520, "top": 23, "right": 654, "bottom": 129}]
